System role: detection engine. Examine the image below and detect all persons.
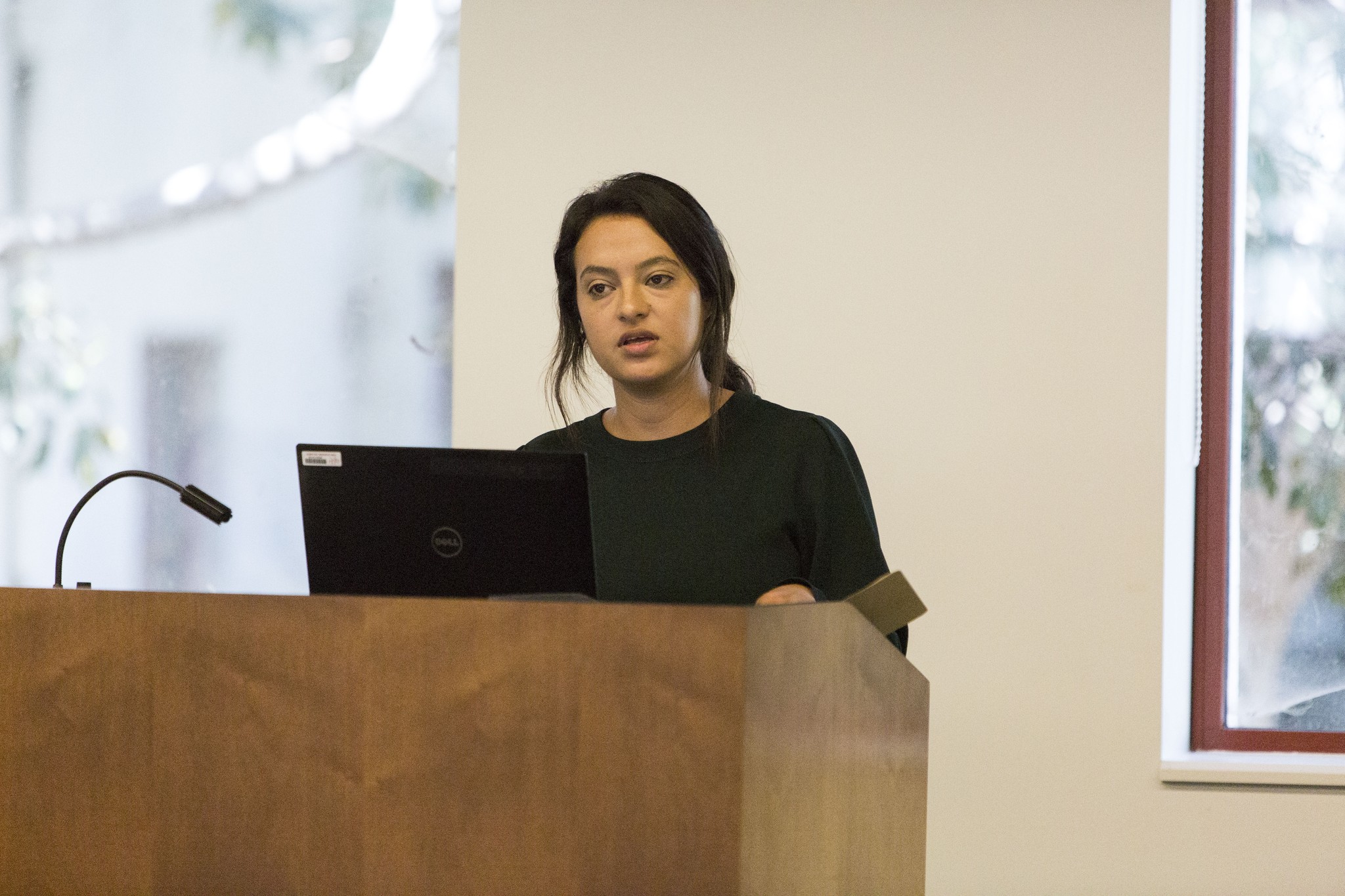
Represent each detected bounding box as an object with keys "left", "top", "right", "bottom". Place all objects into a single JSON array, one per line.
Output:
[{"left": 516, "top": 173, "right": 909, "bottom": 655}]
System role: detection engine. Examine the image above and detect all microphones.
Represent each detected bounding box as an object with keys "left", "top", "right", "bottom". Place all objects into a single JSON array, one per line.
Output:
[{"left": 53, "top": 470, "right": 232, "bottom": 589}]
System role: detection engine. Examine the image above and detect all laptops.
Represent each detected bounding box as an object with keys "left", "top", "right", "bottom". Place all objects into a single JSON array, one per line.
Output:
[{"left": 296, "top": 443, "right": 599, "bottom": 603}]
